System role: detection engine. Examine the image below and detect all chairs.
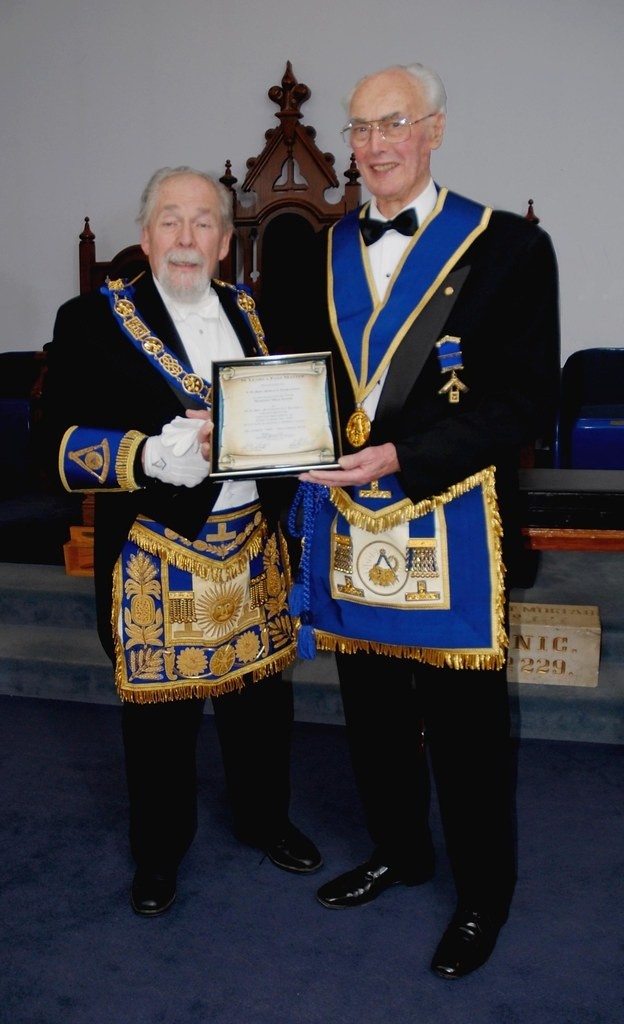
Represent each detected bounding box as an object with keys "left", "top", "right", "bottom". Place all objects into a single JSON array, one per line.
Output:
[{"left": 555, "top": 347, "right": 624, "bottom": 470}]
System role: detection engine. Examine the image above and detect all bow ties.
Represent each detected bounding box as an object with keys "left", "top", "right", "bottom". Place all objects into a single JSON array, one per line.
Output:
[
  {"left": 358, "top": 207, "right": 419, "bottom": 247},
  {"left": 167, "top": 295, "right": 221, "bottom": 323}
]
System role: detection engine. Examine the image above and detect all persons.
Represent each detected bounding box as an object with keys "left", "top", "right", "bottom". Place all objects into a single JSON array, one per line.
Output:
[
  {"left": 20, "top": 165, "right": 323, "bottom": 916},
  {"left": 289, "top": 62, "right": 564, "bottom": 976}
]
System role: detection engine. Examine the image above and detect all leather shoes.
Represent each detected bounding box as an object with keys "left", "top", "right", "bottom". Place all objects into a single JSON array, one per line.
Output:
[
  {"left": 316, "top": 860, "right": 431, "bottom": 909},
  {"left": 430, "top": 905, "right": 501, "bottom": 980},
  {"left": 131, "top": 865, "right": 179, "bottom": 917},
  {"left": 234, "top": 818, "right": 324, "bottom": 875}
]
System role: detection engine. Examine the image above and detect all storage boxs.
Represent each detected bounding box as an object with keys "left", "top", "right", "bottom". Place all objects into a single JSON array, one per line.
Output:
[
  {"left": 572, "top": 416, "right": 623, "bottom": 470},
  {"left": 504, "top": 601, "right": 601, "bottom": 687}
]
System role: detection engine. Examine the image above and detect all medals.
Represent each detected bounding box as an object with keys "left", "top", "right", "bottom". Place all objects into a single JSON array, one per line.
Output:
[{"left": 345, "top": 405, "right": 371, "bottom": 448}]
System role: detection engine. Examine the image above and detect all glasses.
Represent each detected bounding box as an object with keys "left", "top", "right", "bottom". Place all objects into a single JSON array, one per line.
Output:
[{"left": 340, "top": 111, "right": 439, "bottom": 149}]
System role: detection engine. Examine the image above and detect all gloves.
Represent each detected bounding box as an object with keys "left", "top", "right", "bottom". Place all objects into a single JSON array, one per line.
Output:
[
  {"left": 162, "top": 417, "right": 208, "bottom": 458},
  {"left": 144, "top": 416, "right": 210, "bottom": 487}
]
